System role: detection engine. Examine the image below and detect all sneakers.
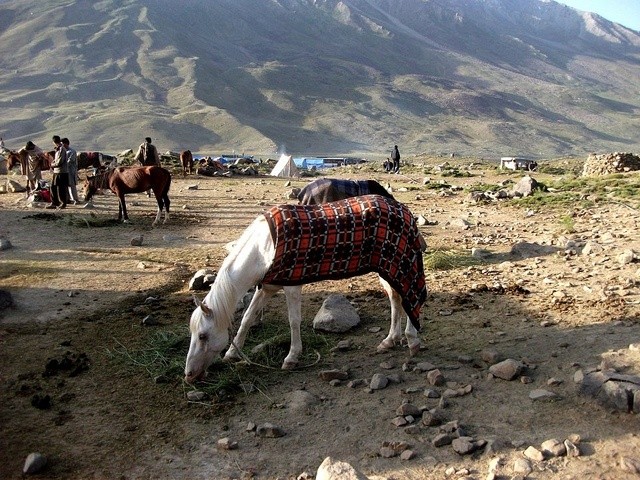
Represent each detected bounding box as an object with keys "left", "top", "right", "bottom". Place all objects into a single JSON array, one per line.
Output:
[
  {"left": 59, "top": 203, "right": 67, "bottom": 209},
  {"left": 46, "top": 204, "right": 56, "bottom": 209}
]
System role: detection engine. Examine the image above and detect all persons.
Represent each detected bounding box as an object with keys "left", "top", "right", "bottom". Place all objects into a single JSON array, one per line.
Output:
[
  {"left": 383, "top": 158, "right": 393, "bottom": 171},
  {"left": 46, "top": 135, "right": 67, "bottom": 209},
  {"left": 134, "top": 136, "right": 161, "bottom": 197},
  {"left": 0, "top": 138, "right": 5, "bottom": 150},
  {"left": 9, "top": 141, "right": 52, "bottom": 199},
  {"left": 391, "top": 145, "right": 400, "bottom": 171},
  {"left": 60, "top": 138, "right": 79, "bottom": 205},
  {"left": 512, "top": 158, "right": 537, "bottom": 172}
]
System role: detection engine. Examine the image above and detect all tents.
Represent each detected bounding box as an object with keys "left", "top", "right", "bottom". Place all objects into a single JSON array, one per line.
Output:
[{"left": 270, "top": 153, "right": 299, "bottom": 178}]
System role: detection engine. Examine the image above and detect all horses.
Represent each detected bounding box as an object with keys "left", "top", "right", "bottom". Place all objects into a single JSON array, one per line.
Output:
[
  {"left": 75, "top": 150, "right": 118, "bottom": 169},
  {"left": 7, "top": 149, "right": 60, "bottom": 178},
  {"left": 181, "top": 150, "right": 196, "bottom": 174},
  {"left": 81, "top": 165, "right": 172, "bottom": 227},
  {"left": 184, "top": 194, "right": 427, "bottom": 383}
]
